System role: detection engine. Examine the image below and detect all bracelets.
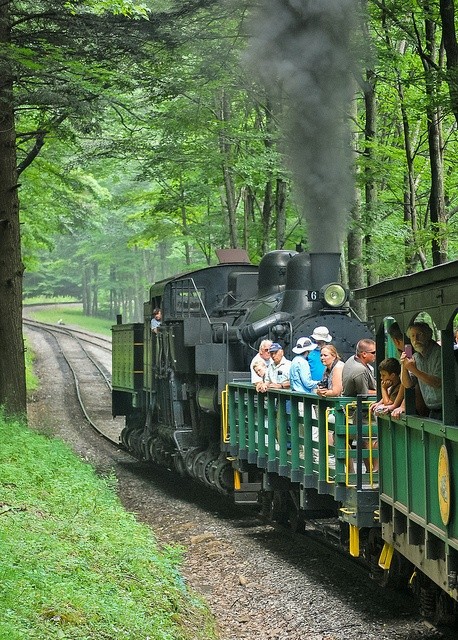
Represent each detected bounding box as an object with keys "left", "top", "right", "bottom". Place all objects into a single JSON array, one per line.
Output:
[{"left": 278, "top": 383, "right": 284, "bottom": 389}]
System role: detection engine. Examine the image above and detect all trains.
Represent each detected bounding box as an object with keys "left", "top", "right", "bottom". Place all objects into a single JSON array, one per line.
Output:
[{"left": 112, "top": 248, "right": 458, "bottom": 640}]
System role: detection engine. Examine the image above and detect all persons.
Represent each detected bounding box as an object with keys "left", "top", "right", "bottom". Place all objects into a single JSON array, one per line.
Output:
[
  {"left": 316, "top": 345, "right": 356, "bottom": 473},
  {"left": 453, "top": 327, "right": 458, "bottom": 350},
  {"left": 252, "top": 360, "right": 269, "bottom": 410},
  {"left": 369, "top": 357, "right": 401, "bottom": 413},
  {"left": 304, "top": 326, "right": 332, "bottom": 393},
  {"left": 399, "top": 321, "right": 442, "bottom": 420},
  {"left": 289, "top": 337, "right": 320, "bottom": 464},
  {"left": 256, "top": 343, "right": 292, "bottom": 450},
  {"left": 250, "top": 340, "right": 273, "bottom": 386},
  {"left": 342, "top": 339, "right": 380, "bottom": 473},
  {"left": 151, "top": 308, "right": 161, "bottom": 334},
  {"left": 382, "top": 322, "right": 431, "bottom": 420}
]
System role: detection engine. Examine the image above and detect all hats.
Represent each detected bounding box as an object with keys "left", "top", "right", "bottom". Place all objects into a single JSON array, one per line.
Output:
[
  {"left": 268, "top": 343, "right": 282, "bottom": 352},
  {"left": 310, "top": 326, "right": 332, "bottom": 342},
  {"left": 291, "top": 337, "right": 318, "bottom": 354}
]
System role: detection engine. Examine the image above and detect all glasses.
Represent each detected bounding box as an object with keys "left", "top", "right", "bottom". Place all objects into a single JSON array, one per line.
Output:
[{"left": 366, "top": 351, "right": 376, "bottom": 354}]
